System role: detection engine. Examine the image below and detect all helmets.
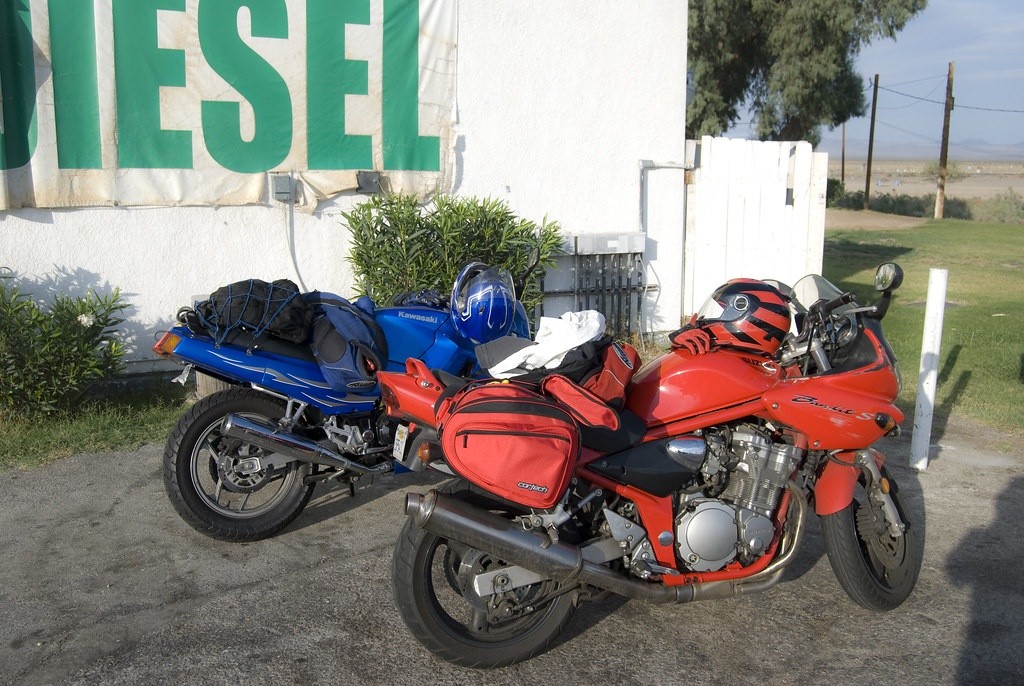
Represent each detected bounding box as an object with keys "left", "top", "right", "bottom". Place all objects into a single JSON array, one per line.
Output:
[
  {"left": 449, "top": 259, "right": 516, "bottom": 348},
  {"left": 693, "top": 277, "right": 791, "bottom": 356}
]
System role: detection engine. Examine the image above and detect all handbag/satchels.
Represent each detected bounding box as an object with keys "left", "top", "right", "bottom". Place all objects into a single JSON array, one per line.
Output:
[
  {"left": 438, "top": 378, "right": 581, "bottom": 511},
  {"left": 301, "top": 290, "right": 388, "bottom": 393}
]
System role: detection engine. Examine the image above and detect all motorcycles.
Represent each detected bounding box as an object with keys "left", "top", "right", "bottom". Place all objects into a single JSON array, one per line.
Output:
[
  {"left": 152, "top": 246, "right": 541, "bottom": 542},
  {"left": 374, "top": 260, "right": 915, "bottom": 670}
]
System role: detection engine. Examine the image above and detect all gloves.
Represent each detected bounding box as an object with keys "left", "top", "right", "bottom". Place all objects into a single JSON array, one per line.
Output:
[
  {"left": 668, "top": 325, "right": 710, "bottom": 355},
  {"left": 391, "top": 288, "right": 449, "bottom": 307}
]
